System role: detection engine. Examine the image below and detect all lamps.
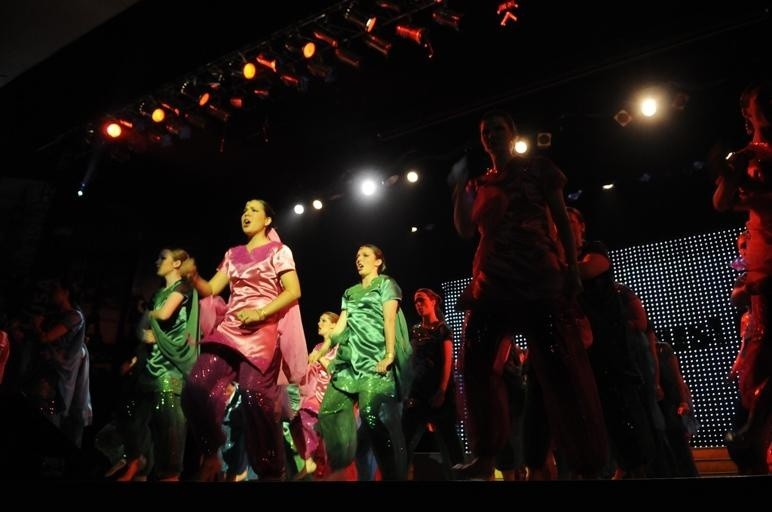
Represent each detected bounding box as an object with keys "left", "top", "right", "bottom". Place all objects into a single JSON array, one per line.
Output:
[{"left": 134, "top": 1, "right": 523, "bottom": 147}]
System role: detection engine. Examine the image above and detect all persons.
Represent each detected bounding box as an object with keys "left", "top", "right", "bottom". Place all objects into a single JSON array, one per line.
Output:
[
  {"left": 560, "top": 207, "right": 695, "bottom": 481},
  {"left": 720, "top": 232, "right": 771, "bottom": 471},
  {"left": 114, "top": 200, "right": 462, "bottom": 484},
  {"left": 450, "top": 106, "right": 614, "bottom": 479},
  {"left": 711, "top": 86, "right": 770, "bottom": 416},
  {"left": 27, "top": 281, "right": 93, "bottom": 475}
]
